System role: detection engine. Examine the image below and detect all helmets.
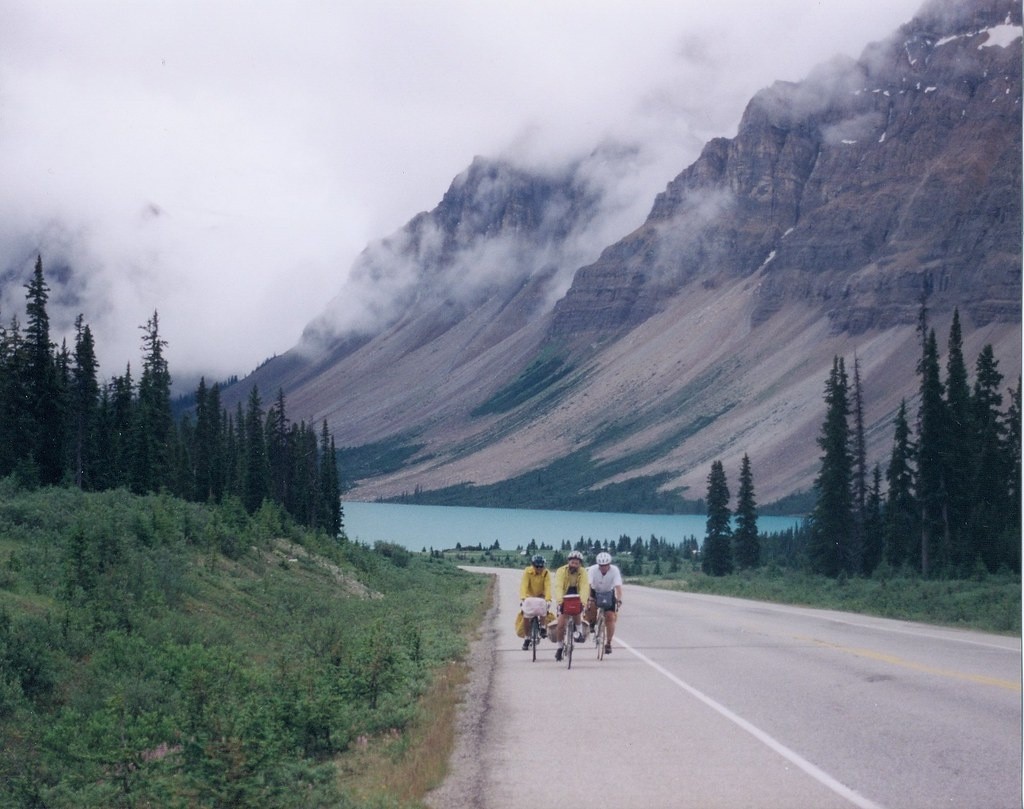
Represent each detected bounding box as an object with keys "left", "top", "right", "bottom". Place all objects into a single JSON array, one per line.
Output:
[
  {"left": 532, "top": 554, "right": 545, "bottom": 567},
  {"left": 568, "top": 551, "right": 583, "bottom": 560},
  {"left": 596, "top": 552, "right": 612, "bottom": 565}
]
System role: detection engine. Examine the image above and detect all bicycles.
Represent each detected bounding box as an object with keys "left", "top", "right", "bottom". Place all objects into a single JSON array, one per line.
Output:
[
  {"left": 520, "top": 598, "right": 550, "bottom": 662},
  {"left": 557, "top": 602, "right": 584, "bottom": 669},
  {"left": 590, "top": 600, "right": 618, "bottom": 662}
]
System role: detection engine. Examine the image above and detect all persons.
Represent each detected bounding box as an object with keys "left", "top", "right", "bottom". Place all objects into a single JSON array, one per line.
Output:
[
  {"left": 555, "top": 550, "right": 591, "bottom": 659},
  {"left": 586, "top": 552, "right": 623, "bottom": 654},
  {"left": 521, "top": 555, "right": 552, "bottom": 650}
]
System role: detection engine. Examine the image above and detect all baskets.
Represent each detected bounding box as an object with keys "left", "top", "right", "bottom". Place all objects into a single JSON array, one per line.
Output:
[
  {"left": 562, "top": 594, "right": 582, "bottom": 615},
  {"left": 595, "top": 591, "right": 614, "bottom": 609}
]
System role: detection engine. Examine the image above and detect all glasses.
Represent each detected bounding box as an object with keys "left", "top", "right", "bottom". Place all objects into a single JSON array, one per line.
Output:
[{"left": 535, "top": 566, "right": 543, "bottom": 568}]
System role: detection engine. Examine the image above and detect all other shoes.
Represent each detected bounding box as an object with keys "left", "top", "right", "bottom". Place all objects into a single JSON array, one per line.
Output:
[
  {"left": 605, "top": 644, "right": 611, "bottom": 653},
  {"left": 578, "top": 632, "right": 585, "bottom": 643},
  {"left": 540, "top": 628, "right": 547, "bottom": 638},
  {"left": 590, "top": 622, "right": 595, "bottom": 633},
  {"left": 555, "top": 647, "right": 563, "bottom": 661},
  {"left": 522, "top": 639, "right": 531, "bottom": 650}
]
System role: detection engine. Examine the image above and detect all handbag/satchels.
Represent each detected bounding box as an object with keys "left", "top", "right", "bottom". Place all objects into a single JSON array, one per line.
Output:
[
  {"left": 574, "top": 618, "right": 590, "bottom": 643},
  {"left": 515, "top": 612, "right": 531, "bottom": 637},
  {"left": 548, "top": 618, "right": 560, "bottom": 643},
  {"left": 546, "top": 612, "right": 556, "bottom": 624}
]
